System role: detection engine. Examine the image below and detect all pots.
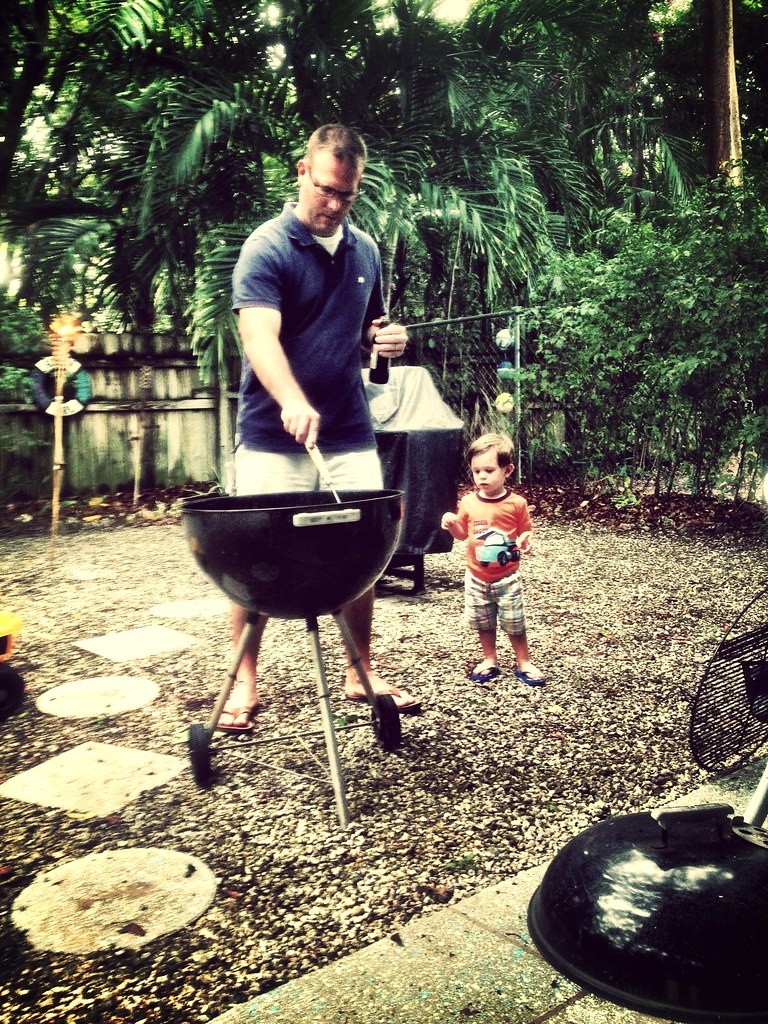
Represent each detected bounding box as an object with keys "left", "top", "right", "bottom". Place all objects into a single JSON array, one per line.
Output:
[
  {"left": 179, "top": 489, "right": 406, "bottom": 620},
  {"left": 527, "top": 803, "right": 768, "bottom": 1024}
]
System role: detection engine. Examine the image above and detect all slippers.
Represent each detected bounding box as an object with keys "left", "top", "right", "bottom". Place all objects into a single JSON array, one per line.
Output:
[
  {"left": 345, "top": 684, "right": 423, "bottom": 712},
  {"left": 216, "top": 702, "right": 261, "bottom": 733},
  {"left": 515, "top": 667, "right": 546, "bottom": 685},
  {"left": 471, "top": 664, "right": 500, "bottom": 680}
]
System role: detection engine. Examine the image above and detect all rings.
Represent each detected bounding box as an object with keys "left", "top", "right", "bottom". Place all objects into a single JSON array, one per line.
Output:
[{"left": 395, "top": 343, "right": 398, "bottom": 350}]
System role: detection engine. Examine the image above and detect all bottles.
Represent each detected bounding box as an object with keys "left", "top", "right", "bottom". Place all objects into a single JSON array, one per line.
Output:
[{"left": 368, "top": 318, "right": 390, "bottom": 384}]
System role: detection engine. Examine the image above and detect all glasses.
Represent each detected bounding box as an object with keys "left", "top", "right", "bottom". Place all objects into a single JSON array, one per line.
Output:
[{"left": 308, "top": 168, "right": 361, "bottom": 202}]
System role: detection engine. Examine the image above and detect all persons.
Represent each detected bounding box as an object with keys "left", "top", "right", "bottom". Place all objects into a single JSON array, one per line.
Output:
[
  {"left": 441, "top": 432, "right": 547, "bottom": 687},
  {"left": 215, "top": 122, "right": 422, "bottom": 730}
]
